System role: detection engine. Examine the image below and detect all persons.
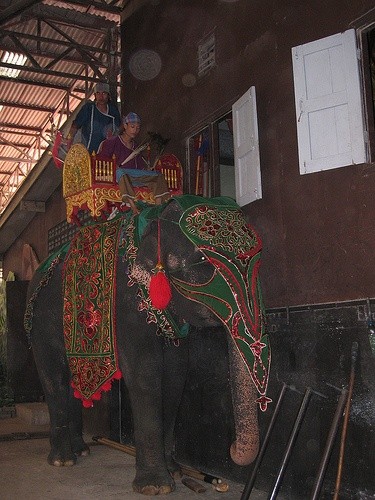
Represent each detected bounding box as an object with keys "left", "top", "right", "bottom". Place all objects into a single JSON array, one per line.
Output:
[
  {"left": 97, "top": 111, "right": 171, "bottom": 216},
  {"left": 68, "top": 82, "right": 122, "bottom": 154}
]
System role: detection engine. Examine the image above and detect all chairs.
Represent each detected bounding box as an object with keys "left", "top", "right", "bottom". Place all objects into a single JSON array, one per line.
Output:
[{"left": 63, "top": 144, "right": 184, "bottom": 223}]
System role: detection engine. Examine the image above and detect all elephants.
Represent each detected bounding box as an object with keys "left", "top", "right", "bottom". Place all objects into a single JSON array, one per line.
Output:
[{"left": 25, "top": 194, "right": 263, "bottom": 496}]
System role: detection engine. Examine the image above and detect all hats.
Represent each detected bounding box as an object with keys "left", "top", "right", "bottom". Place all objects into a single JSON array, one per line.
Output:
[
  {"left": 123, "top": 112, "right": 142, "bottom": 123},
  {"left": 93, "top": 83, "right": 110, "bottom": 94}
]
